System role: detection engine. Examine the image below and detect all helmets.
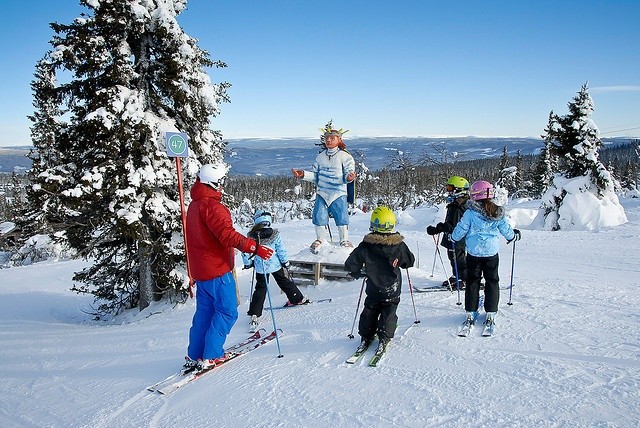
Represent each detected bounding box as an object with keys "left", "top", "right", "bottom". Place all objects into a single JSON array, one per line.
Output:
[
  {"left": 253, "top": 209, "right": 272, "bottom": 227},
  {"left": 369, "top": 207, "right": 395, "bottom": 234},
  {"left": 470, "top": 181, "right": 494, "bottom": 200},
  {"left": 446, "top": 175, "right": 470, "bottom": 202},
  {"left": 200, "top": 164, "right": 228, "bottom": 184}
]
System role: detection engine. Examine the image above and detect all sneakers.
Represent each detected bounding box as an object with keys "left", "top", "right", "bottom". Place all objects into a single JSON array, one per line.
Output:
[
  {"left": 443, "top": 277, "right": 456, "bottom": 285},
  {"left": 286, "top": 300, "right": 306, "bottom": 305},
  {"left": 205, "top": 354, "right": 228, "bottom": 363},
  {"left": 451, "top": 281, "right": 466, "bottom": 288},
  {"left": 466, "top": 311, "right": 477, "bottom": 320},
  {"left": 485, "top": 311, "right": 496, "bottom": 322}
]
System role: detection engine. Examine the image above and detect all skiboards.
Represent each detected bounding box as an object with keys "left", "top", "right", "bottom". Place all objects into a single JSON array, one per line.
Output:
[
  {"left": 458, "top": 295, "right": 496, "bottom": 337},
  {"left": 248, "top": 298, "right": 333, "bottom": 333},
  {"left": 412, "top": 284, "right": 517, "bottom": 292},
  {"left": 346, "top": 338, "right": 392, "bottom": 367},
  {"left": 147, "top": 328, "right": 283, "bottom": 395}
]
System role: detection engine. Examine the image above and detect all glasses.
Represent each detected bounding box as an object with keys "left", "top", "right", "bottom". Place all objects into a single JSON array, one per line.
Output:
[{"left": 447, "top": 184, "right": 468, "bottom": 192}]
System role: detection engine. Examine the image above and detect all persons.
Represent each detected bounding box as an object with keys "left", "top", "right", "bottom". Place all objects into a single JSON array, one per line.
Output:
[
  {"left": 447, "top": 182, "right": 521, "bottom": 323},
  {"left": 184, "top": 162, "right": 274, "bottom": 370},
  {"left": 292, "top": 117, "right": 356, "bottom": 250},
  {"left": 242, "top": 210, "right": 306, "bottom": 330},
  {"left": 427, "top": 176, "right": 471, "bottom": 288},
  {"left": 344, "top": 207, "right": 415, "bottom": 344}
]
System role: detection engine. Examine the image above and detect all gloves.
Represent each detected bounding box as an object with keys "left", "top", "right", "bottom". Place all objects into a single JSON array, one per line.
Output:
[
  {"left": 346, "top": 271, "right": 362, "bottom": 280},
  {"left": 283, "top": 261, "right": 291, "bottom": 270},
  {"left": 427, "top": 225, "right": 436, "bottom": 235},
  {"left": 436, "top": 222, "right": 448, "bottom": 233},
  {"left": 244, "top": 238, "right": 273, "bottom": 260},
  {"left": 514, "top": 229, "right": 521, "bottom": 241}
]
